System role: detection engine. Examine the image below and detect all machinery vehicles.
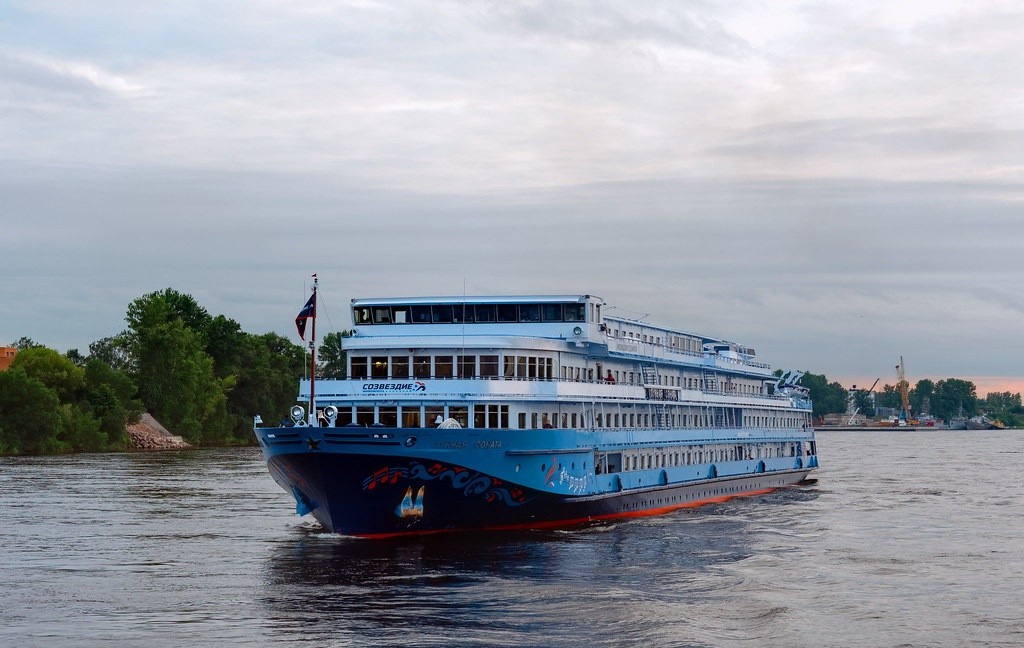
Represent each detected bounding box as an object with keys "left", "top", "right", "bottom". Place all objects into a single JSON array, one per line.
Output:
[
  {"left": 846, "top": 377, "right": 881, "bottom": 426},
  {"left": 894, "top": 356, "right": 922, "bottom": 426}
]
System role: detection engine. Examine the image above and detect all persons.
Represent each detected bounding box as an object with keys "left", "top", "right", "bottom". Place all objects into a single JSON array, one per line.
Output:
[
  {"left": 603, "top": 369, "right": 616, "bottom": 399},
  {"left": 543, "top": 420, "right": 552, "bottom": 429},
  {"left": 595, "top": 464, "right": 601, "bottom": 475}
]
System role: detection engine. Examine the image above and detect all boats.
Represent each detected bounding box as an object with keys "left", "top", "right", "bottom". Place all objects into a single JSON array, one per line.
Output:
[
  {"left": 948, "top": 401, "right": 1010, "bottom": 430},
  {"left": 252, "top": 271, "right": 820, "bottom": 542}
]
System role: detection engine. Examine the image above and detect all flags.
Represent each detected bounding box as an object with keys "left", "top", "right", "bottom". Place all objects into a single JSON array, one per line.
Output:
[{"left": 295, "top": 293, "right": 314, "bottom": 341}]
